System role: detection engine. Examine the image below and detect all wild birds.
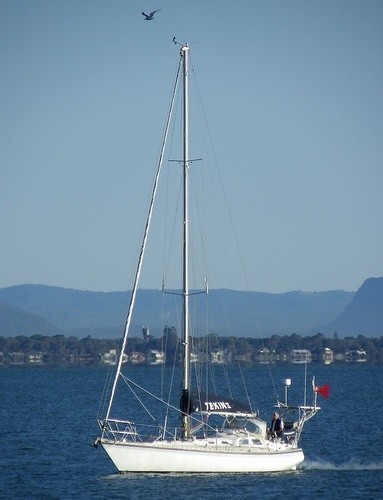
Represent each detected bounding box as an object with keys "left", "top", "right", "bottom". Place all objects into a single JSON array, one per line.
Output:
[{"left": 142, "top": 9, "right": 161, "bottom": 20}]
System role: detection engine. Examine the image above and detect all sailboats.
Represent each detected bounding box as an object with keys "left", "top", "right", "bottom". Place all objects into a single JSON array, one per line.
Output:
[{"left": 92, "top": 41, "right": 321, "bottom": 472}]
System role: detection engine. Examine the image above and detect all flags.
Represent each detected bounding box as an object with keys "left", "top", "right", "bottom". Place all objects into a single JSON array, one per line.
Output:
[{"left": 317, "top": 384, "right": 331, "bottom": 399}]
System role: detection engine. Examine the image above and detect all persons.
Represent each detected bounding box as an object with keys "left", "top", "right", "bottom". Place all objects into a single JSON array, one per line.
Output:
[{"left": 269, "top": 412, "right": 284, "bottom": 440}]
